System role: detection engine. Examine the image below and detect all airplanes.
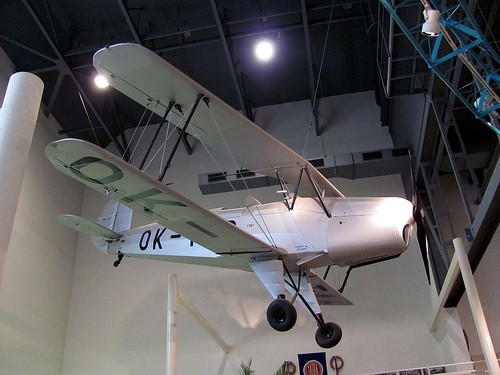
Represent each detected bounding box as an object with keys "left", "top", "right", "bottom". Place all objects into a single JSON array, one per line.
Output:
[{"left": 43, "top": 42, "right": 419, "bottom": 347}]
